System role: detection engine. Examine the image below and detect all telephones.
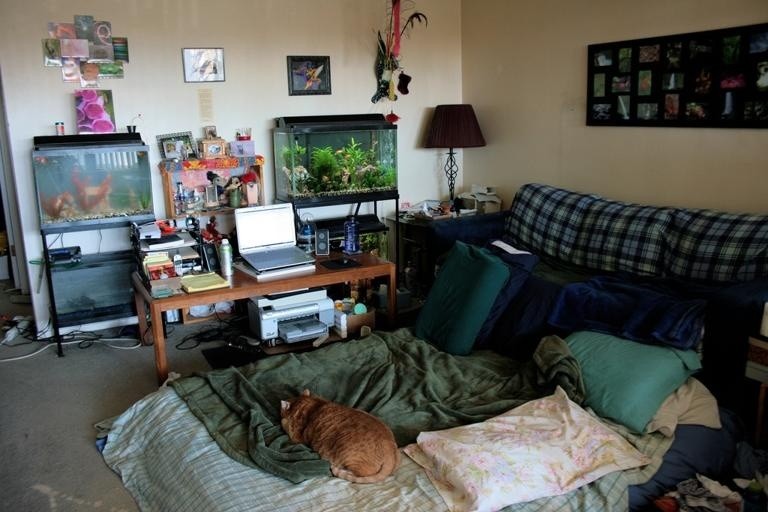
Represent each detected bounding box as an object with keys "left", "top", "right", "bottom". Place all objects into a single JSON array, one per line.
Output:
[{"left": 173, "top": 248, "right": 183, "bottom": 276}]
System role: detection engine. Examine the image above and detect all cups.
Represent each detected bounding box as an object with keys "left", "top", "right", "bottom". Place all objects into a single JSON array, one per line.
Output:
[
  {"left": 238, "top": 128, "right": 253, "bottom": 140},
  {"left": 126, "top": 125, "right": 136, "bottom": 133},
  {"left": 229, "top": 188, "right": 240, "bottom": 208}
]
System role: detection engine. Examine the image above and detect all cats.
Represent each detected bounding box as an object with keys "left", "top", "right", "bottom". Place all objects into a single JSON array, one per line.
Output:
[{"left": 281, "top": 388, "right": 400, "bottom": 484}]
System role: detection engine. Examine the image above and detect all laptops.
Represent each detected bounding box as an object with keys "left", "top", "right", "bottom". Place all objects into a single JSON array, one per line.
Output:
[{"left": 235, "top": 203, "right": 317, "bottom": 272}]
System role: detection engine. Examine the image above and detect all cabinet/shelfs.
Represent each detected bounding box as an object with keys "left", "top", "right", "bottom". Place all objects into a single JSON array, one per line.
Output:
[
  {"left": 157, "top": 154, "right": 267, "bottom": 325},
  {"left": 276, "top": 195, "right": 400, "bottom": 289}
]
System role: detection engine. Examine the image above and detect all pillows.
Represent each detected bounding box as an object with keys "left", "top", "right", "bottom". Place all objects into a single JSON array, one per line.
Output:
[{"left": 399, "top": 384, "right": 656, "bottom": 512}]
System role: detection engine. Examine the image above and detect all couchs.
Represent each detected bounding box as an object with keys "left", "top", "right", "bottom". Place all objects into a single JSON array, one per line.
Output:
[{"left": 413, "top": 183, "right": 767, "bottom": 405}]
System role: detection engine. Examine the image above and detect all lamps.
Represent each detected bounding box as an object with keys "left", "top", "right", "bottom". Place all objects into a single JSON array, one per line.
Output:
[{"left": 419, "top": 101, "right": 485, "bottom": 206}]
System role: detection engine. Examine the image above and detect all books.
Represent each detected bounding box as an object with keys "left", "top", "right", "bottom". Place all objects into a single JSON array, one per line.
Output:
[{"left": 139, "top": 221, "right": 229, "bottom": 298}]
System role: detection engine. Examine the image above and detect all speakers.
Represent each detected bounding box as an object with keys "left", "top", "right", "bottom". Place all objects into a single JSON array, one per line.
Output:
[
  {"left": 202, "top": 242, "right": 220, "bottom": 272},
  {"left": 315, "top": 230, "right": 330, "bottom": 256}
]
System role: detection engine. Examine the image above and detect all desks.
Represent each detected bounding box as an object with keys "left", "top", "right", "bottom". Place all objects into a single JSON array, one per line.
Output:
[
  {"left": 385, "top": 206, "right": 515, "bottom": 302},
  {"left": 126, "top": 252, "right": 397, "bottom": 387},
  {"left": 38, "top": 225, "right": 170, "bottom": 358}
]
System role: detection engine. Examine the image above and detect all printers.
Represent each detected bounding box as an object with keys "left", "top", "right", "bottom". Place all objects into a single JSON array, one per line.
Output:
[{"left": 248, "top": 288, "right": 334, "bottom": 344}]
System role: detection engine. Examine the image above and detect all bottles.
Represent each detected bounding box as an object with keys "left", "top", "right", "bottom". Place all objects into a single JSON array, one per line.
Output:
[
  {"left": 54, "top": 122, "right": 65, "bottom": 136},
  {"left": 334, "top": 295, "right": 367, "bottom": 315},
  {"left": 220, "top": 239, "right": 233, "bottom": 276},
  {"left": 344, "top": 214, "right": 360, "bottom": 252},
  {"left": 171, "top": 181, "right": 206, "bottom": 216},
  {"left": 297, "top": 224, "right": 316, "bottom": 252}
]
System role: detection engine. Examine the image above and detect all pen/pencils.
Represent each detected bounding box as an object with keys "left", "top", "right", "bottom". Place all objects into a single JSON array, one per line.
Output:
[{"left": 235, "top": 126, "right": 252, "bottom": 138}]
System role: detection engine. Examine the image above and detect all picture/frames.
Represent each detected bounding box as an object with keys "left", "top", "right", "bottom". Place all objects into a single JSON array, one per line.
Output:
[
  {"left": 181, "top": 46, "right": 227, "bottom": 84},
  {"left": 154, "top": 125, "right": 227, "bottom": 163},
  {"left": 286, "top": 53, "right": 332, "bottom": 97},
  {"left": 584, "top": 21, "right": 768, "bottom": 129}
]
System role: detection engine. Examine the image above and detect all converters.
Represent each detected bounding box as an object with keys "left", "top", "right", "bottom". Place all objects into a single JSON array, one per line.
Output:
[
  {"left": 13, "top": 316, "right": 24, "bottom": 320},
  {"left": 6, "top": 320, "right": 17, "bottom": 327}
]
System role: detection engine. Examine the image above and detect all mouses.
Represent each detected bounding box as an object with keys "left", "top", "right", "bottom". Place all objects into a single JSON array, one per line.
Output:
[{"left": 340, "top": 257, "right": 350, "bottom": 264}]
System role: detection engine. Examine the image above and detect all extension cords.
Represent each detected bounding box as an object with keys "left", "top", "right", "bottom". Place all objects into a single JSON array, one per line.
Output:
[{"left": 4, "top": 315, "right": 32, "bottom": 343}]
